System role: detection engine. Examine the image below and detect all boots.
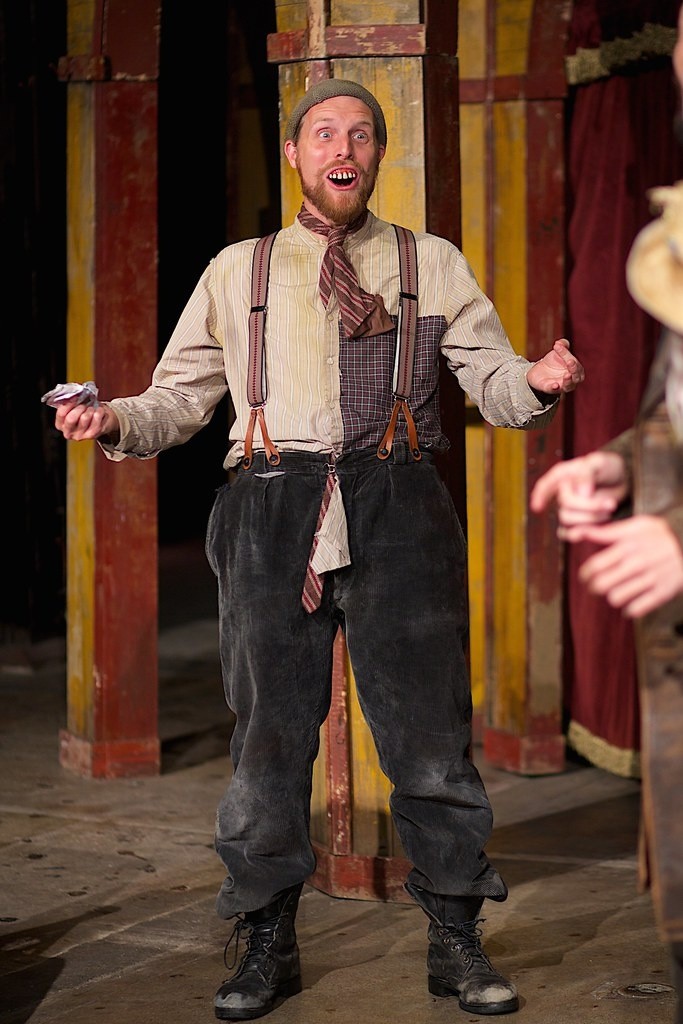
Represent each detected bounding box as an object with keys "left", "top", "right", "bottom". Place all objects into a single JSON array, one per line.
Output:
[
  {"left": 213, "top": 881, "right": 302, "bottom": 1019},
  {"left": 403, "top": 882, "right": 519, "bottom": 1014}
]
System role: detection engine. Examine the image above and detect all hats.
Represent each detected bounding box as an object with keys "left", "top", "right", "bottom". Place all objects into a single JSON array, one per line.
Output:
[{"left": 285, "top": 79, "right": 387, "bottom": 152}]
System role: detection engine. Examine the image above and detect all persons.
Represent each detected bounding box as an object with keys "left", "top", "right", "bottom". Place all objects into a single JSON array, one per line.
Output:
[
  {"left": 529, "top": 3, "right": 683, "bottom": 1024},
  {"left": 52, "top": 80, "right": 586, "bottom": 1020}
]
